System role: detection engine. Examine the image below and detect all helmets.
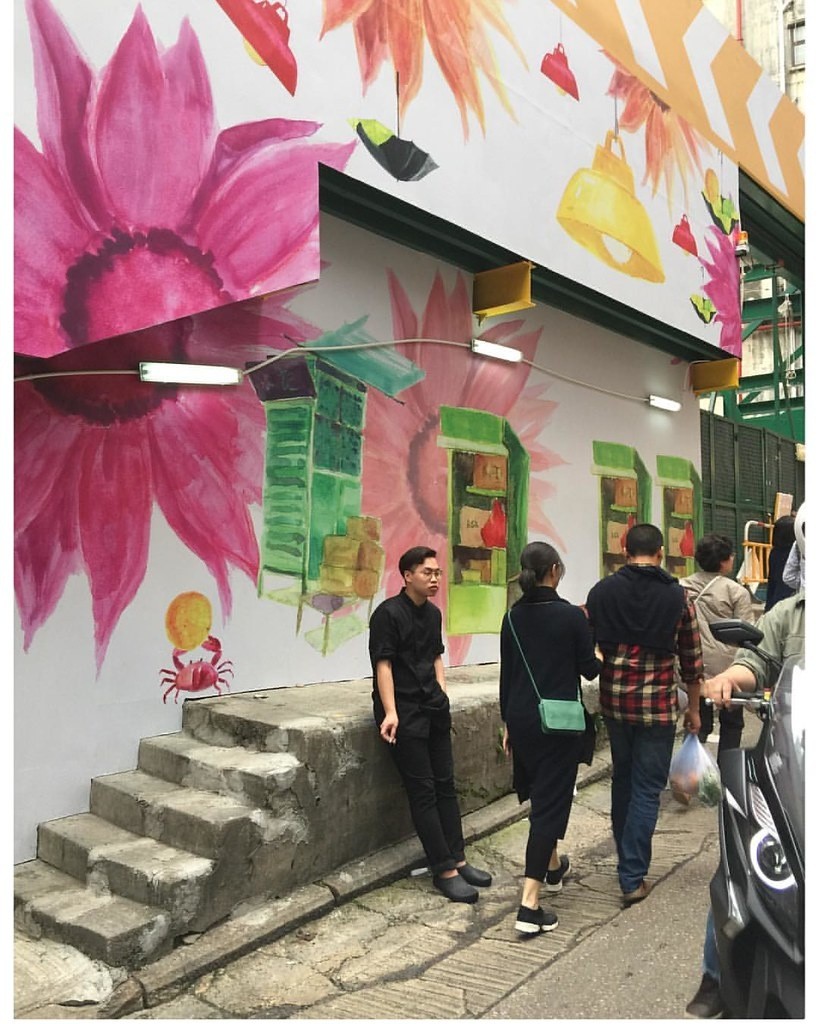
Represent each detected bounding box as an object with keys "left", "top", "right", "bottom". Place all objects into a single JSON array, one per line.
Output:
[{"left": 794, "top": 501, "right": 804, "bottom": 561}]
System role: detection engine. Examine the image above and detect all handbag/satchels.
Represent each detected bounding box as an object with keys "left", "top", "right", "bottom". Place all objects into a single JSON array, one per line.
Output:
[
  {"left": 669, "top": 732, "right": 724, "bottom": 807},
  {"left": 538, "top": 699, "right": 586, "bottom": 735}
]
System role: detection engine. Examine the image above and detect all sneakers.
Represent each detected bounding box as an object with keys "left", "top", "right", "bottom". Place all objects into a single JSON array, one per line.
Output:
[
  {"left": 515, "top": 905, "right": 558, "bottom": 933},
  {"left": 544, "top": 855, "right": 571, "bottom": 892}
]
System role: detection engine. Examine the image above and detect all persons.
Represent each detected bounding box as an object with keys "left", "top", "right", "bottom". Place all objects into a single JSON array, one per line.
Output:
[
  {"left": 764, "top": 515, "right": 801, "bottom": 615},
  {"left": 501, "top": 542, "right": 604, "bottom": 934},
  {"left": 678, "top": 533, "right": 755, "bottom": 771},
  {"left": 702, "top": 500, "right": 805, "bottom": 729},
  {"left": 586, "top": 523, "right": 702, "bottom": 905},
  {"left": 368, "top": 545, "right": 492, "bottom": 903}
]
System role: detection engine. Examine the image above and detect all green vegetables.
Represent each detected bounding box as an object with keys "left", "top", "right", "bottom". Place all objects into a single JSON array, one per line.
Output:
[{"left": 697, "top": 770, "right": 720, "bottom": 808}]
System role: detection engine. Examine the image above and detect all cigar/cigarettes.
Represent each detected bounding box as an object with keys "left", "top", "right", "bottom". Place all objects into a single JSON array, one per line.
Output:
[{"left": 388, "top": 737, "right": 392, "bottom": 743}]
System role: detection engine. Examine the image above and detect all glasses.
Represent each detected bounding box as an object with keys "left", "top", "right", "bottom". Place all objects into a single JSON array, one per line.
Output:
[
  {"left": 730, "top": 551, "right": 737, "bottom": 558},
  {"left": 413, "top": 568, "right": 443, "bottom": 581}
]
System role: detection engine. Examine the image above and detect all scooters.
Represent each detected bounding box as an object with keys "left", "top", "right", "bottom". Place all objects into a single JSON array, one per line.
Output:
[{"left": 704, "top": 618, "right": 806, "bottom": 1019}]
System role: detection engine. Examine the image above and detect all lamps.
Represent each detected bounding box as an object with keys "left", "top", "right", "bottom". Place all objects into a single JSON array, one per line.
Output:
[
  {"left": 649, "top": 395, "right": 682, "bottom": 413},
  {"left": 471, "top": 337, "right": 525, "bottom": 364},
  {"left": 139, "top": 360, "right": 243, "bottom": 387}
]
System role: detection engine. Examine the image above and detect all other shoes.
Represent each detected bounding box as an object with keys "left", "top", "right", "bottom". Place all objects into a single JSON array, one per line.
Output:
[
  {"left": 623, "top": 878, "right": 646, "bottom": 900},
  {"left": 673, "top": 790, "right": 691, "bottom": 804},
  {"left": 455, "top": 860, "right": 491, "bottom": 886},
  {"left": 434, "top": 871, "right": 479, "bottom": 902}
]
